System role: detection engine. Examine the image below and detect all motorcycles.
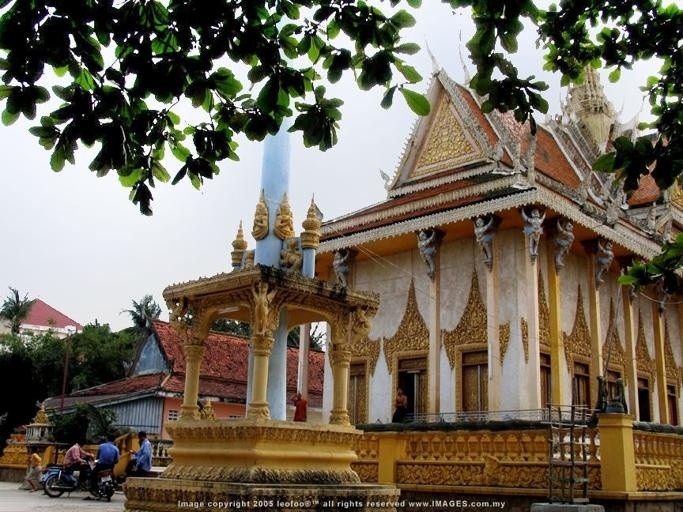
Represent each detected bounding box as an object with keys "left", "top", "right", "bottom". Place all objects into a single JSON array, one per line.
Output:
[{"left": 38, "top": 455, "right": 160, "bottom": 502}]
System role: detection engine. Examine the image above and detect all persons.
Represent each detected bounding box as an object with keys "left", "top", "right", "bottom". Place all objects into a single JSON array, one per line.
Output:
[
  {"left": 333, "top": 249, "right": 350, "bottom": 287},
  {"left": 474, "top": 214, "right": 494, "bottom": 265},
  {"left": 276, "top": 203, "right": 293, "bottom": 231},
  {"left": 24, "top": 445, "right": 43, "bottom": 492},
  {"left": 521, "top": 208, "right": 547, "bottom": 256},
  {"left": 555, "top": 221, "right": 574, "bottom": 268},
  {"left": 89, "top": 433, "right": 120, "bottom": 489},
  {"left": 393, "top": 388, "right": 408, "bottom": 422},
  {"left": 596, "top": 239, "right": 614, "bottom": 284},
  {"left": 253, "top": 204, "right": 267, "bottom": 231},
  {"left": 125, "top": 431, "right": 152, "bottom": 477},
  {"left": 64, "top": 435, "right": 95, "bottom": 490},
  {"left": 290, "top": 392, "right": 308, "bottom": 421},
  {"left": 417, "top": 229, "right": 436, "bottom": 277}
]
